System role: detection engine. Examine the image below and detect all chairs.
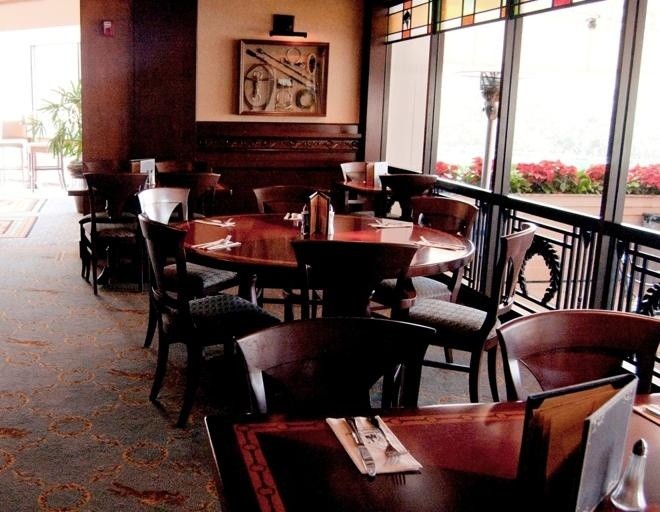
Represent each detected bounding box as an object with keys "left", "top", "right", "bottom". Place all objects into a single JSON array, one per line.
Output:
[
  {"left": 1, "top": 121, "right": 28, "bottom": 171},
  {"left": 26, "top": 118, "right": 65, "bottom": 192},
  {"left": 67, "top": 152, "right": 660, "bottom": 427}
]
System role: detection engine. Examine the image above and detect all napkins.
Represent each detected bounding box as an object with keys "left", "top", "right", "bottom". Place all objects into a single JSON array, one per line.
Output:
[{"left": 325, "top": 416, "right": 422, "bottom": 474}]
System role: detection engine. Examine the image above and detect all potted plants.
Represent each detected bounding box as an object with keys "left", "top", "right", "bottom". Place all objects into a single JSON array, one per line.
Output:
[{"left": 35, "top": 74, "right": 86, "bottom": 216}]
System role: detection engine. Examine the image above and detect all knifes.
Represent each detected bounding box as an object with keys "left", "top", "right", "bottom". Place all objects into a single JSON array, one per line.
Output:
[{"left": 345, "top": 415, "right": 376, "bottom": 476}]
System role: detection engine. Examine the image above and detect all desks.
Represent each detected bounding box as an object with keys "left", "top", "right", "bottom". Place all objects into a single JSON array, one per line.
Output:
[{"left": 202, "top": 394, "right": 659, "bottom": 512}]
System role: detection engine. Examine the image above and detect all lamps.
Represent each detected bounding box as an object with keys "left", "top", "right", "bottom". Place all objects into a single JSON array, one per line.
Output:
[{"left": 267, "top": 12, "right": 309, "bottom": 39}]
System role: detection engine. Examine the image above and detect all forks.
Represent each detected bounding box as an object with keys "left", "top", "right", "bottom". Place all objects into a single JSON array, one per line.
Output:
[
  {"left": 391, "top": 473, "right": 406, "bottom": 488},
  {"left": 366, "top": 415, "right": 410, "bottom": 457}
]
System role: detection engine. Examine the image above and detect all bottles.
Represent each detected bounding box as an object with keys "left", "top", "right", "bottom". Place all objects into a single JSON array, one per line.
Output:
[
  {"left": 300, "top": 204, "right": 310, "bottom": 234},
  {"left": 328, "top": 204, "right": 335, "bottom": 234},
  {"left": 150, "top": 169, "right": 156, "bottom": 187},
  {"left": 607, "top": 437, "right": 650, "bottom": 512}
]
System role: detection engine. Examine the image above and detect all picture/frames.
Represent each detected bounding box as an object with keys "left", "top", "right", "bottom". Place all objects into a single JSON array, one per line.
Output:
[{"left": 231, "top": 36, "right": 331, "bottom": 120}]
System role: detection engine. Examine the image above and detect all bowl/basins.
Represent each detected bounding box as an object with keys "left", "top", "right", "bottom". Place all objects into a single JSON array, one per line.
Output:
[
  {"left": 189, "top": 173, "right": 220, "bottom": 190},
  {"left": 83, "top": 160, "right": 111, "bottom": 172}
]
